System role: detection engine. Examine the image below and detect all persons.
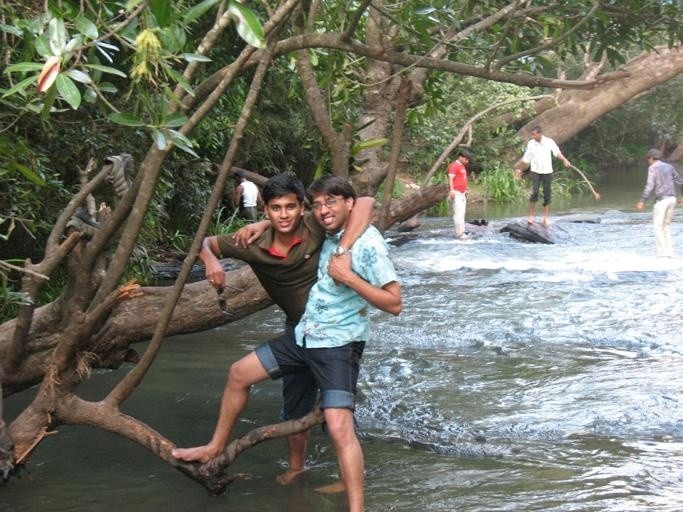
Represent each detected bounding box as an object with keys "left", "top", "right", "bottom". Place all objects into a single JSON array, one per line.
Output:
[
  {"left": 636, "top": 148, "right": 683, "bottom": 258},
  {"left": 447, "top": 150, "right": 472, "bottom": 239},
  {"left": 170, "top": 176, "right": 403, "bottom": 512},
  {"left": 513, "top": 125, "right": 571, "bottom": 226},
  {"left": 233, "top": 169, "right": 266, "bottom": 225},
  {"left": 201, "top": 173, "right": 377, "bottom": 469}
]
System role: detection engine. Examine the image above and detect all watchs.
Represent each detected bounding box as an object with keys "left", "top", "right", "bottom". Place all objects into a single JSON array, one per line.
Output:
[{"left": 335, "top": 246, "right": 345, "bottom": 256}]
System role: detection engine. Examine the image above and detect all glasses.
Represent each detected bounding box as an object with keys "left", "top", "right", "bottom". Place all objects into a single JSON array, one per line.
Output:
[
  {"left": 215, "top": 285, "right": 245, "bottom": 321},
  {"left": 312, "top": 197, "right": 345, "bottom": 209}
]
